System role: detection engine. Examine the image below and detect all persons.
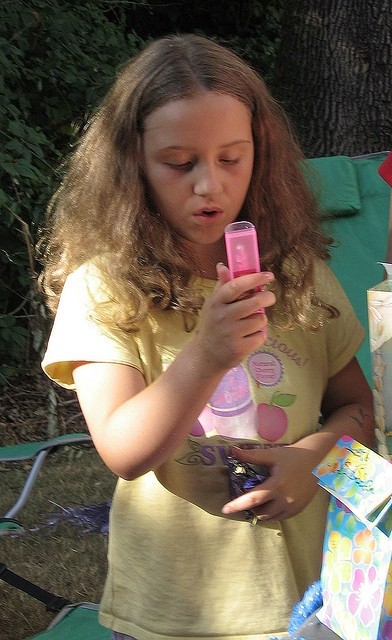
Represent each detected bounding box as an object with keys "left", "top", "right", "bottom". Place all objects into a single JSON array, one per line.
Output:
[{"left": 38, "top": 32, "right": 376, "bottom": 640}]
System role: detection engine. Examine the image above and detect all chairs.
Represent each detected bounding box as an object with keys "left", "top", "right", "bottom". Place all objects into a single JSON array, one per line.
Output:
[
  {"left": 0, "top": 432, "right": 115, "bottom": 639},
  {"left": 297, "top": 151, "right": 392, "bottom": 460}
]
